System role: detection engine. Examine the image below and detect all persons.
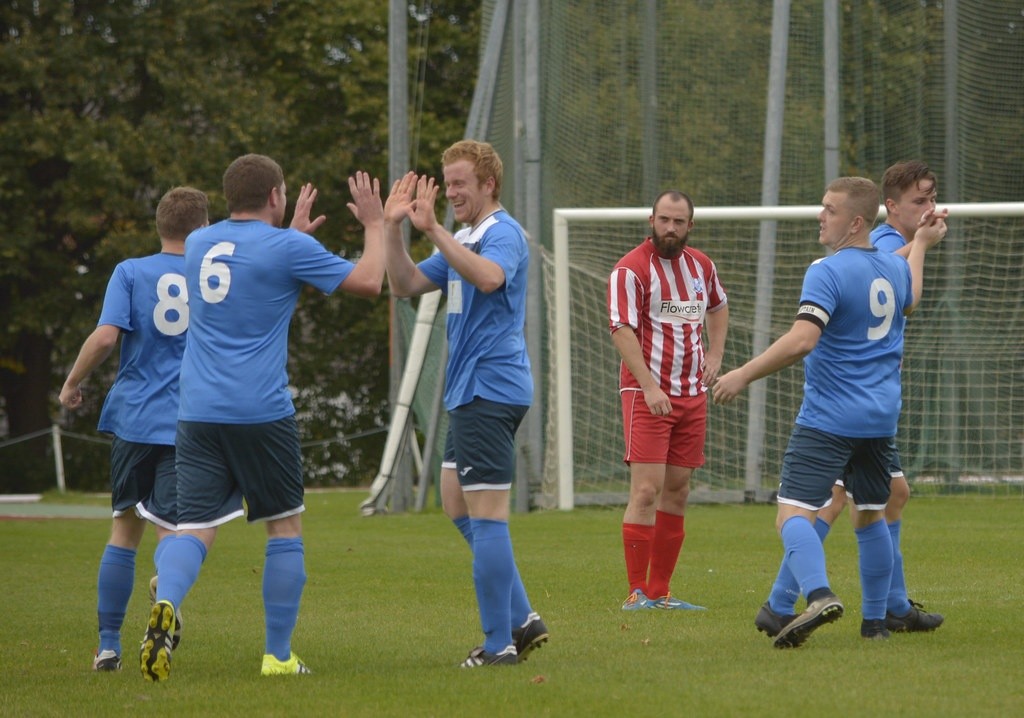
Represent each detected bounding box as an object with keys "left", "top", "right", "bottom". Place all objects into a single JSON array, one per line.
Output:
[
  {"left": 609, "top": 190, "right": 728, "bottom": 611},
  {"left": 386, "top": 140, "right": 550, "bottom": 671},
  {"left": 58, "top": 186, "right": 208, "bottom": 672},
  {"left": 713, "top": 177, "right": 946, "bottom": 649},
  {"left": 754, "top": 160, "right": 947, "bottom": 637},
  {"left": 141, "top": 154, "right": 386, "bottom": 681}
]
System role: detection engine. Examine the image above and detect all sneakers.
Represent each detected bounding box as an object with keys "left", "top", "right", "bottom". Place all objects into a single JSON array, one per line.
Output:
[
  {"left": 260, "top": 651, "right": 312, "bottom": 674},
  {"left": 509, "top": 612, "right": 548, "bottom": 663},
  {"left": 92, "top": 650, "right": 123, "bottom": 671},
  {"left": 859, "top": 618, "right": 890, "bottom": 640},
  {"left": 459, "top": 643, "right": 518, "bottom": 667},
  {"left": 138, "top": 599, "right": 176, "bottom": 681},
  {"left": 621, "top": 589, "right": 670, "bottom": 610},
  {"left": 660, "top": 595, "right": 705, "bottom": 610},
  {"left": 773, "top": 594, "right": 844, "bottom": 649},
  {"left": 149, "top": 576, "right": 182, "bottom": 649},
  {"left": 885, "top": 599, "right": 944, "bottom": 631},
  {"left": 753, "top": 600, "right": 796, "bottom": 638}
]
